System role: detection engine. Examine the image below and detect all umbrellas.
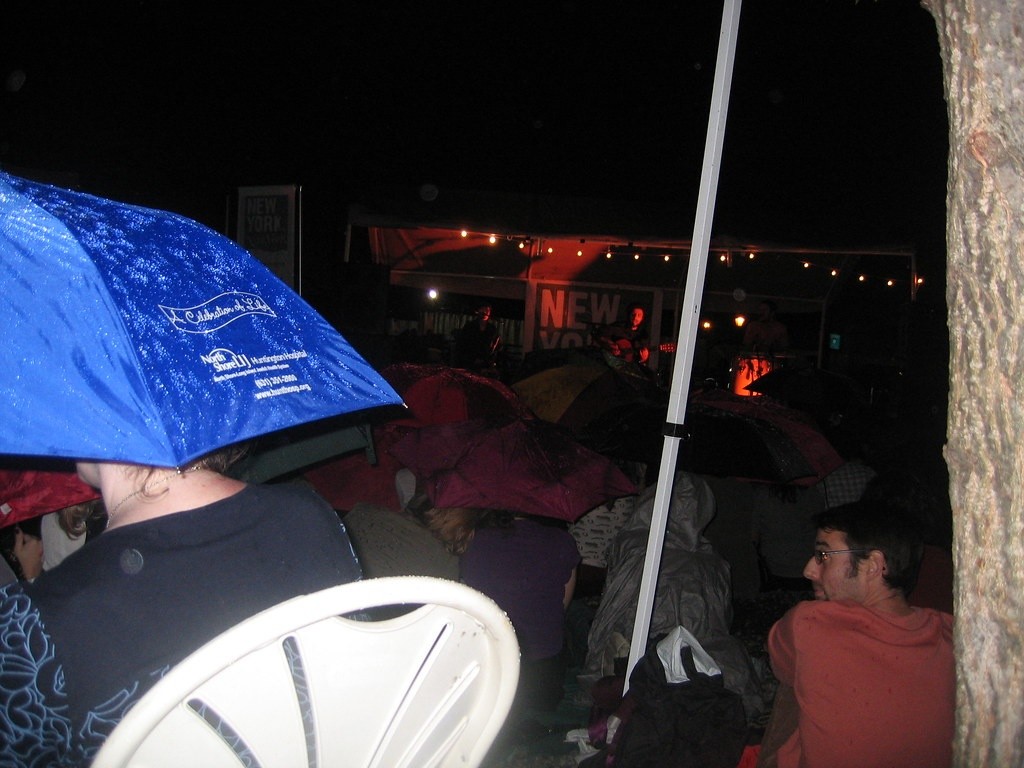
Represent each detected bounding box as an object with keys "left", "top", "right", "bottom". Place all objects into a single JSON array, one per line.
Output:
[
  {"left": 237, "top": 351, "right": 847, "bottom": 524},
  {"left": 0, "top": 169, "right": 409, "bottom": 469}
]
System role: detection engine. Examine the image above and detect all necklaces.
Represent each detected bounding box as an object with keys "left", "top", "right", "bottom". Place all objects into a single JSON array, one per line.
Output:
[{"left": 104, "top": 465, "right": 205, "bottom": 527}]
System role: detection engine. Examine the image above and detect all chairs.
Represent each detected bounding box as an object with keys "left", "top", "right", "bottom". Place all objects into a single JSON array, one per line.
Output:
[{"left": 88, "top": 576, "right": 522, "bottom": 768}]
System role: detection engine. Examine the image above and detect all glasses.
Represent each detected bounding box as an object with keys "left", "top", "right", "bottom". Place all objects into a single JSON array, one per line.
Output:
[{"left": 814, "top": 549, "right": 859, "bottom": 563}]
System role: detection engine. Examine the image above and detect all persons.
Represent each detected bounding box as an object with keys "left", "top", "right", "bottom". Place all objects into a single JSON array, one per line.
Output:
[
  {"left": 571, "top": 496, "right": 633, "bottom": 599},
  {"left": 742, "top": 301, "right": 792, "bottom": 352},
  {"left": 599, "top": 304, "right": 652, "bottom": 363},
  {"left": 588, "top": 468, "right": 737, "bottom": 675},
  {"left": 341, "top": 491, "right": 477, "bottom": 586},
  {"left": 453, "top": 507, "right": 580, "bottom": 711},
  {"left": 764, "top": 502, "right": 953, "bottom": 767},
  {"left": 458, "top": 302, "right": 506, "bottom": 376},
  {"left": 0, "top": 451, "right": 364, "bottom": 768}
]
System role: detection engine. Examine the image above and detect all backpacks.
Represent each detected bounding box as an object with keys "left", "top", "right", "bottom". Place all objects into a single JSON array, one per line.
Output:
[{"left": 617, "top": 626, "right": 750, "bottom": 768}]
